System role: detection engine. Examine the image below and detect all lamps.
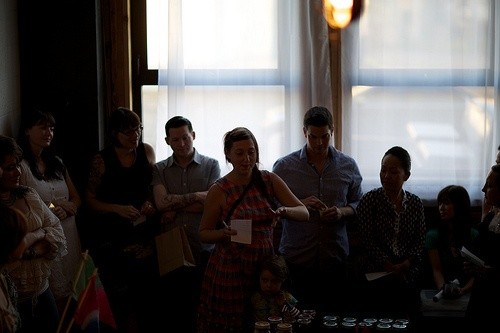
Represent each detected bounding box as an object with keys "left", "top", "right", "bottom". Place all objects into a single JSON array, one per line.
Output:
[{"left": 321, "top": 0, "right": 354, "bottom": 29}]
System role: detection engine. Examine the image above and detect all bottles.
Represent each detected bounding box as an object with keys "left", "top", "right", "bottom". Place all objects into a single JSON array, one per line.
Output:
[{"left": 253, "top": 315, "right": 294, "bottom": 333}]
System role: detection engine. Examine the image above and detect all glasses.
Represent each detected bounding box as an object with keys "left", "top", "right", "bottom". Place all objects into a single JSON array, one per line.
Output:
[{"left": 117, "top": 124, "right": 143, "bottom": 136}]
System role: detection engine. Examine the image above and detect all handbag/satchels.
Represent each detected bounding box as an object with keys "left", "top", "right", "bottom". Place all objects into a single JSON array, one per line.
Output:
[{"left": 154, "top": 226, "right": 196, "bottom": 276}]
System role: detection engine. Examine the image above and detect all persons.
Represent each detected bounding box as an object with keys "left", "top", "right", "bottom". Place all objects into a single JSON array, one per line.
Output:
[
  {"left": 0, "top": 103, "right": 223, "bottom": 332},
  {"left": 270, "top": 106, "right": 364, "bottom": 304},
  {"left": 196, "top": 126, "right": 310, "bottom": 333},
  {"left": 248, "top": 254, "right": 299, "bottom": 323},
  {"left": 353, "top": 145, "right": 430, "bottom": 310},
  {"left": 429, "top": 184, "right": 487, "bottom": 298},
  {"left": 456, "top": 144, "right": 500, "bottom": 332}
]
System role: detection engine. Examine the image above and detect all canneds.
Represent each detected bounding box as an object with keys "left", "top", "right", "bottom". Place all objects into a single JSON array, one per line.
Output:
[
  {"left": 254, "top": 316, "right": 293, "bottom": 333},
  {"left": 321, "top": 315, "right": 410, "bottom": 333}
]
